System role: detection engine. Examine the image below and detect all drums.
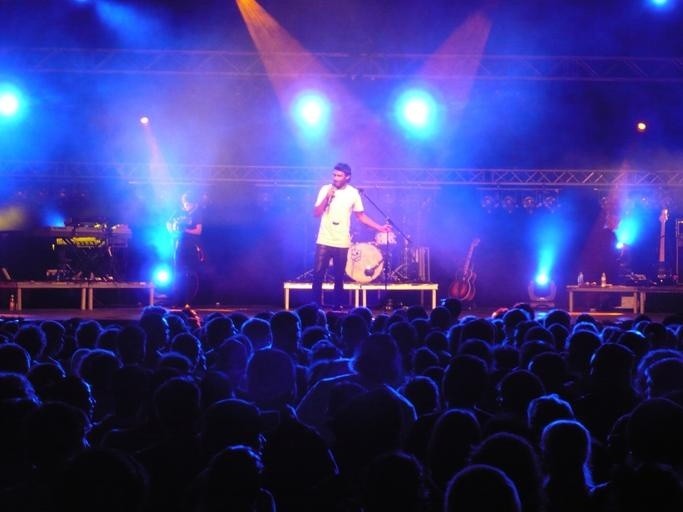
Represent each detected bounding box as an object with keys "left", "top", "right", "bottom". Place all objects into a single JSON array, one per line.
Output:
[
  {"left": 375, "top": 231, "right": 397, "bottom": 245},
  {"left": 345, "top": 242, "right": 384, "bottom": 283}
]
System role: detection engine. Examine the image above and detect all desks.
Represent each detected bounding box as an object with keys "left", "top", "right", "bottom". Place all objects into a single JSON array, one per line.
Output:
[
  {"left": 4, "top": 279, "right": 154, "bottom": 314},
  {"left": 280, "top": 279, "right": 438, "bottom": 312},
  {"left": 568, "top": 283, "right": 683, "bottom": 315}
]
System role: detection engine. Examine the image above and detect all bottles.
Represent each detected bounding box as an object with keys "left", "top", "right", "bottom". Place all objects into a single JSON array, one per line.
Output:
[
  {"left": 600, "top": 272, "right": 608, "bottom": 289},
  {"left": 576, "top": 271, "right": 585, "bottom": 287},
  {"left": 8, "top": 294, "right": 15, "bottom": 312}
]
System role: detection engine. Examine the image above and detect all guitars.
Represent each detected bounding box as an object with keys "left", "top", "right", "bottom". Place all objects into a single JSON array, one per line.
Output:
[
  {"left": 449, "top": 238, "right": 480, "bottom": 301},
  {"left": 651, "top": 207, "right": 673, "bottom": 286}
]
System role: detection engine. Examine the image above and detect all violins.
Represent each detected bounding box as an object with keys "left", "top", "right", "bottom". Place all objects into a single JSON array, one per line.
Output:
[{"left": 169, "top": 216, "right": 194, "bottom": 230}]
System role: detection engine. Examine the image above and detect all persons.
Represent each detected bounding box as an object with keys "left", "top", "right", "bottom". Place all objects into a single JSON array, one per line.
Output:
[
  {"left": 166, "top": 191, "right": 202, "bottom": 309},
  {"left": 311, "top": 163, "right": 393, "bottom": 311}
]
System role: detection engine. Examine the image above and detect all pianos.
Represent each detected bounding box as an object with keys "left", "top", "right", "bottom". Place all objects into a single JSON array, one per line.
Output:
[{"left": 27, "top": 226, "right": 131, "bottom": 239}]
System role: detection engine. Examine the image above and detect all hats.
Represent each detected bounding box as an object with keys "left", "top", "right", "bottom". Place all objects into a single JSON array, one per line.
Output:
[{"left": 334, "top": 162, "right": 352, "bottom": 183}]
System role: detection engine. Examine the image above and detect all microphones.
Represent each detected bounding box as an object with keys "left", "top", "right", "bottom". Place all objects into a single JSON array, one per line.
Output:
[
  {"left": 327, "top": 181, "right": 336, "bottom": 205},
  {"left": 364, "top": 268, "right": 375, "bottom": 277}
]
